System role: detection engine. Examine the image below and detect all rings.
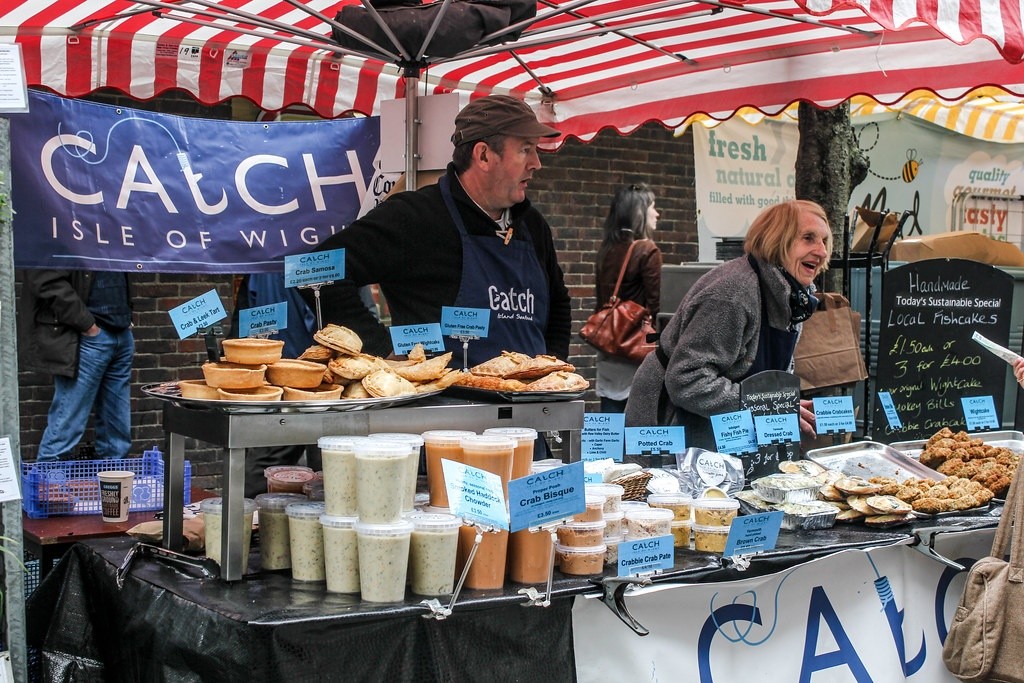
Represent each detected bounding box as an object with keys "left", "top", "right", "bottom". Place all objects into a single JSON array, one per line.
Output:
[{"left": 1016, "top": 379, "right": 1023, "bottom": 383}]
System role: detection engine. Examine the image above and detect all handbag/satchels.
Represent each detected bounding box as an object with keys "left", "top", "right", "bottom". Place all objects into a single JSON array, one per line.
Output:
[
  {"left": 792, "top": 294, "right": 869, "bottom": 396},
  {"left": 848, "top": 205, "right": 898, "bottom": 252},
  {"left": 942, "top": 555, "right": 1024, "bottom": 683},
  {"left": 579, "top": 299, "right": 657, "bottom": 363}
]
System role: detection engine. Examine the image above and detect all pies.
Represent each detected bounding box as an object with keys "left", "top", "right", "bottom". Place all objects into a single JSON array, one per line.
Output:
[
  {"left": 871, "top": 427, "right": 1023, "bottom": 516},
  {"left": 179, "top": 323, "right": 590, "bottom": 403},
  {"left": 782, "top": 457, "right": 916, "bottom": 525}
]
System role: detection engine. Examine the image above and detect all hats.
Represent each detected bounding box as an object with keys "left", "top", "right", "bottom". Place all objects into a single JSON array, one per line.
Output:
[{"left": 450, "top": 95, "right": 562, "bottom": 148}]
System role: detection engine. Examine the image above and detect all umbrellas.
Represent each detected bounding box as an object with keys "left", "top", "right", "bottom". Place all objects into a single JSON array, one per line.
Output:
[{"left": 0, "top": 0, "right": 1024, "bottom": 191}]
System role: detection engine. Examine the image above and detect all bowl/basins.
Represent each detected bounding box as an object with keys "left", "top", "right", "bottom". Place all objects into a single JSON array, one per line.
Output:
[
  {"left": 555, "top": 484, "right": 740, "bottom": 575},
  {"left": 38, "top": 480, "right": 101, "bottom": 512}
]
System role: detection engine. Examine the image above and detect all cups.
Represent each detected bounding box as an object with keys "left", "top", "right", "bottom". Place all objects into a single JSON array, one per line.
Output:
[
  {"left": 200, "top": 427, "right": 552, "bottom": 603},
  {"left": 97, "top": 470, "right": 136, "bottom": 522}
]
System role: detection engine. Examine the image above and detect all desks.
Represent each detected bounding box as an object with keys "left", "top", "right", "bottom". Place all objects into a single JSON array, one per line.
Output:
[
  {"left": 160, "top": 397, "right": 587, "bottom": 580},
  {"left": 18, "top": 480, "right": 218, "bottom": 589}
]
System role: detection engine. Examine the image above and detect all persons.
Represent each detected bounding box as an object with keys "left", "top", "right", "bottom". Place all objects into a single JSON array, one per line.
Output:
[
  {"left": 1013, "top": 357, "right": 1024, "bottom": 389},
  {"left": 294, "top": 95, "right": 572, "bottom": 475},
  {"left": 622, "top": 200, "right": 833, "bottom": 469},
  {"left": 15, "top": 268, "right": 135, "bottom": 474},
  {"left": 594, "top": 183, "right": 662, "bottom": 413},
  {"left": 221, "top": 270, "right": 319, "bottom": 500}
]
